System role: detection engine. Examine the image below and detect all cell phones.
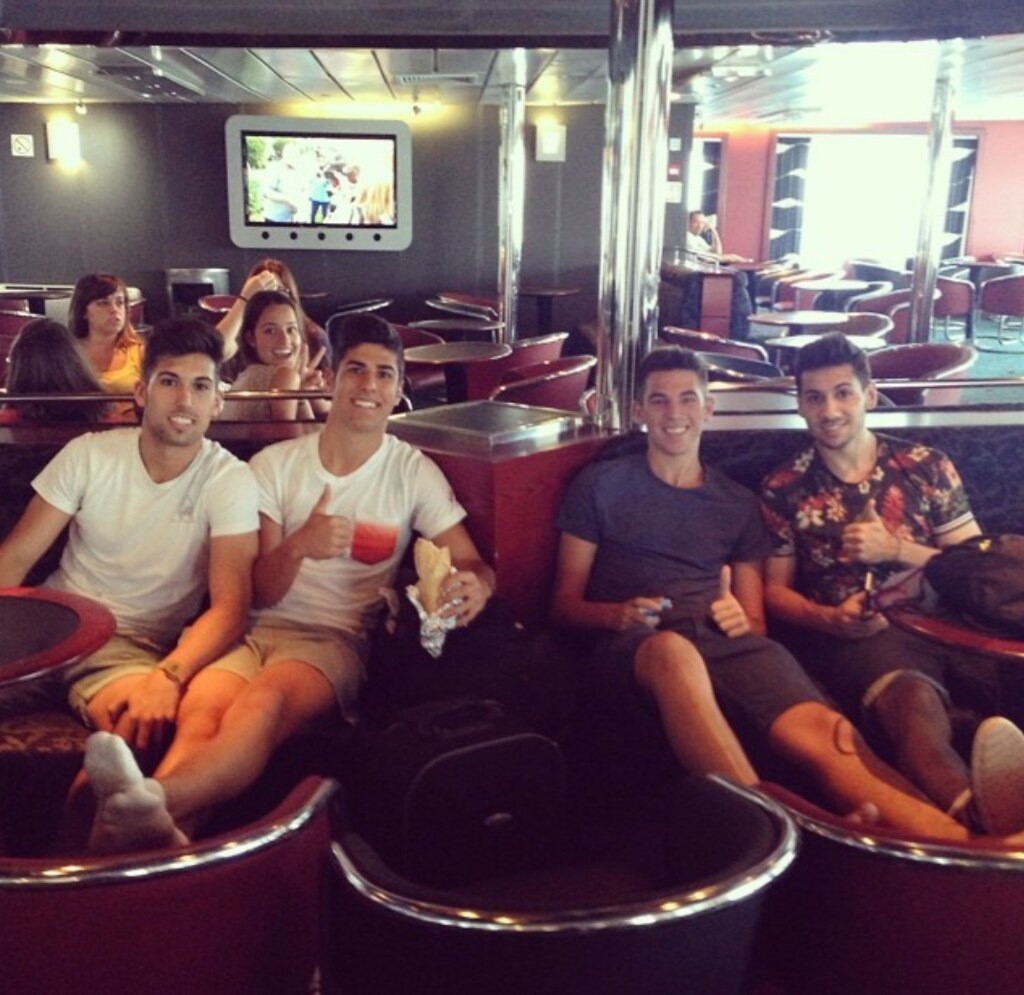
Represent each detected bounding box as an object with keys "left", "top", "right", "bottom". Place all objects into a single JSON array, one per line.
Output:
[{"left": 864, "top": 571, "right": 875, "bottom": 611}]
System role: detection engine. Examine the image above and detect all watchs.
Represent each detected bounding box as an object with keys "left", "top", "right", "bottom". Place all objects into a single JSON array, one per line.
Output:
[{"left": 156, "top": 659, "right": 190, "bottom": 693}]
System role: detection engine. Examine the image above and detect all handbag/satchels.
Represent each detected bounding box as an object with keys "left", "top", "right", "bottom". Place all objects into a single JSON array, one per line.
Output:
[{"left": 920, "top": 530, "right": 1023, "bottom": 631}]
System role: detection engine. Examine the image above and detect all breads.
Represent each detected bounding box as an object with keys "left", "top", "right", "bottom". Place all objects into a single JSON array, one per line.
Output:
[{"left": 413, "top": 537, "right": 451, "bottom": 616}]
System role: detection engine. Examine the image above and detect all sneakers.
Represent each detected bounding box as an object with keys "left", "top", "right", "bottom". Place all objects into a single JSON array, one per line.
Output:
[{"left": 965, "top": 715, "right": 1024, "bottom": 835}]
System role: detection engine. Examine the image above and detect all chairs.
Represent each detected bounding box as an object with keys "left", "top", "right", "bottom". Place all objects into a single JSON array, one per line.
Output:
[{"left": 0, "top": 720, "right": 1023, "bottom": 995}]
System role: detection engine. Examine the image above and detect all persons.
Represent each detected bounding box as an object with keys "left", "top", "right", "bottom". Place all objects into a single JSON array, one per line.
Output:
[
  {"left": 253, "top": 143, "right": 389, "bottom": 229},
  {"left": 0, "top": 317, "right": 119, "bottom": 431},
  {"left": 216, "top": 288, "right": 326, "bottom": 424},
  {"left": 755, "top": 330, "right": 1023, "bottom": 839},
  {"left": 686, "top": 210, "right": 754, "bottom": 267},
  {"left": 0, "top": 311, "right": 264, "bottom": 838},
  {"left": 83, "top": 312, "right": 501, "bottom": 855},
  {"left": 207, "top": 257, "right": 338, "bottom": 419},
  {"left": 65, "top": 272, "right": 149, "bottom": 425},
  {"left": 547, "top": 347, "right": 1023, "bottom": 856}
]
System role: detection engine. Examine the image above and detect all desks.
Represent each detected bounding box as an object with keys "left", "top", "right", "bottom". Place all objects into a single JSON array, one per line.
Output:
[
  {"left": 1, "top": 580, "right": 121, "bottom": 715},
  {"left": 884, "top": 594, "right": 1023, "bottom": 716},
  {"left": 1, "top": 229, "right": 1024, "bottom": 456}
]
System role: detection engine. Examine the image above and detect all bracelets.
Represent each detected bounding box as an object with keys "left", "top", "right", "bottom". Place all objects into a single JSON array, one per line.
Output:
[
  {"left": 891, "top": 535, "right": 902, "bottom": 562},
  {"left": 237, "top": 294, "right": 249, "bottom": 302}
]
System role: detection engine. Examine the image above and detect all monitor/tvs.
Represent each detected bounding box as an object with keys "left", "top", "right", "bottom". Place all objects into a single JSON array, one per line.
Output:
[{"left": 224, "top": 114, "right": 414, "bottom": 252}]
